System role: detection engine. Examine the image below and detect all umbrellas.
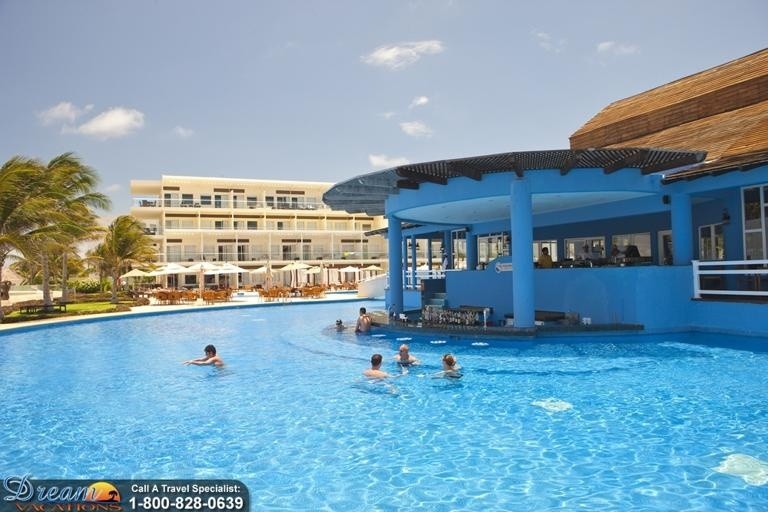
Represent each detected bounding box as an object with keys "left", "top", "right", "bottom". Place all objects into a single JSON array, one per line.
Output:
[{"left": 118, "top": 259, "right": 384, "bottom": 298}]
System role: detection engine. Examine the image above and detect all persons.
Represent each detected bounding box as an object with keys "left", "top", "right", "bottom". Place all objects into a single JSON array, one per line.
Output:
[
  {"left": 181, "top": 344, "right": 222, "bottom": 367},
  {"left": 538, "top": 245, "right": 553, "bottom": 268},
  {"left": 580, "top": 243, "right": 591, "bottom": 260},
  {"left": 354, "top": 307, "right": 373, "bottom": 334},
  {"left": 334, "top": 319, "right": 346, "bottom": 329},
  {"left": 430, "top": 352, "right": 462, "bottom": 377},
  {"left": 394, "top": 345, "right": 421, "bottom": 366},
  {"left": 362, "top": 354, "right": 409, "bottom": 395}
]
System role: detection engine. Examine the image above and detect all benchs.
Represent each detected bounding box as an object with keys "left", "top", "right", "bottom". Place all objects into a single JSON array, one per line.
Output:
[
  {"left": 443, "top": 305, "right": 493, "bottom": 314},
  {"left": 504, "top": 310, "right": 564, "bottom": 327},
  {"left": 19, "top": 304, "right": 67, "bottom": 313}
]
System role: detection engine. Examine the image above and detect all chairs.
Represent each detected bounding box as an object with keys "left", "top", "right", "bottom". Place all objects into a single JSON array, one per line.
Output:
[{"left": 154, "top": 282, "right": 358, "bottom": 305}]
